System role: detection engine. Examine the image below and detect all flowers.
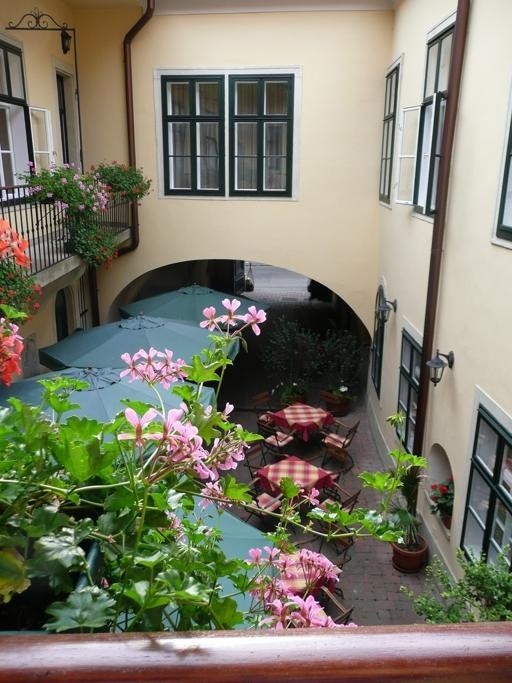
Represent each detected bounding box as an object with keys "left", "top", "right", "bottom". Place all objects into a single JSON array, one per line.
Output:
[
  {"left": 430, "top": 476, "right": 454, "bottom": 517},
  {"left": 0, "top": 217, "right": 43, "bottom": 387},
  {"left": 13, "top": 158, "right": 153, "bottom": 269},
  {"left": 1, "top": 294, "right": 427, "bottom": 628}
]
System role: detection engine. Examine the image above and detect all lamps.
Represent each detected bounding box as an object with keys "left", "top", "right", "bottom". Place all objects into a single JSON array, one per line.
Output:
[
  {"left": 378, "top": 298, "right": 397, "bottom": 324},
  {"left": 4, "top": 8, "right": 76, "bottom": 54},
  {"left": 425, "top": 349, "right": 455, "bottom": 387}
]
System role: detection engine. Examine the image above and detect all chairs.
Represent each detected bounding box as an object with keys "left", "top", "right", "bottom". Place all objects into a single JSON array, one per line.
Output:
[
  {"left": 319, "top": 420, "right": 361, "bottom": 556},
  {"left": 242, "top": 392, "right": 296, "bottom": 529}
]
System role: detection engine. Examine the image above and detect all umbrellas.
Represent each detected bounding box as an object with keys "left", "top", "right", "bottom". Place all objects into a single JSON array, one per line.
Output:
[
  {"left": 0, "top": 366, "right": 218, "bottom": 466},
  {"left": 123, "top": 281, "right": 272, "bottom": 328},
  {"left": 38, "top": 304, "right": 245, "bottom": 374},
  {"left": 0, "top": 480, "right": 286, "bottom": 633}
]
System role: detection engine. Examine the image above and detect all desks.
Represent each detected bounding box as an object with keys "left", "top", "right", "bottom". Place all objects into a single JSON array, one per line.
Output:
[{"left": 255, "top": 400, "right": 331, "bottom": 502}]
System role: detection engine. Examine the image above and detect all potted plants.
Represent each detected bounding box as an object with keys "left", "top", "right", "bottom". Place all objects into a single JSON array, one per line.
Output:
[{"left": 388, "top": 466, "right": 427, "bottom": 574}]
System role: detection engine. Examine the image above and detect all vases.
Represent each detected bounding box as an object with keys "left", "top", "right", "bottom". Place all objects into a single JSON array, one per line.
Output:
[
  {"left": 2, "top": 499, "right": 109, "bottom": 634},
  {"left": 441, "top": 514, "right": 450, "bottom": 530}
]
